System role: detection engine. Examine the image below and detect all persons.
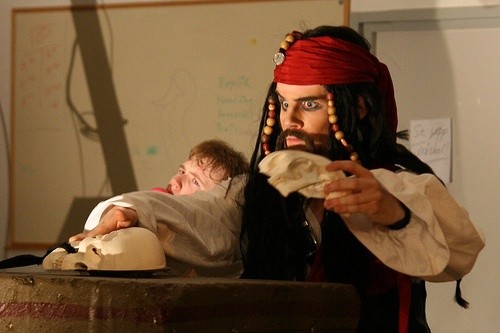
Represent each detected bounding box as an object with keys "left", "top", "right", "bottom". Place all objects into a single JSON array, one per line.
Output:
[
  {"left": 157, "top": 136, "right": 251, "bottom": 202},
  {"left": 67, "top": 23, "right": 487, "bottom": 333}
]
